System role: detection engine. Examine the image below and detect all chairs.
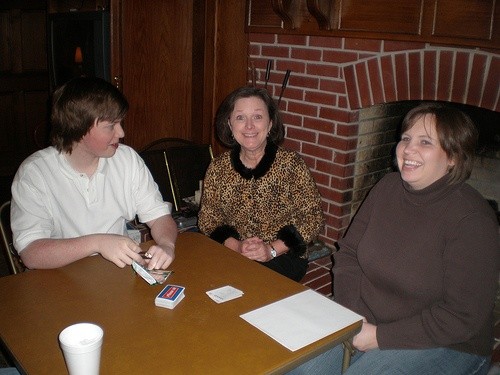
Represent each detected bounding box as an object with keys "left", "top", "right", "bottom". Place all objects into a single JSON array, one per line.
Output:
[{"left": 137, "top": 136, "right": 214, "bottom": 216}]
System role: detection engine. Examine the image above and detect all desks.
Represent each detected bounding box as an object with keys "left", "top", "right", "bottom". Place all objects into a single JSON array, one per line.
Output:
[{"left": 0, "top": 231, "right": 363, "bottom": 375}]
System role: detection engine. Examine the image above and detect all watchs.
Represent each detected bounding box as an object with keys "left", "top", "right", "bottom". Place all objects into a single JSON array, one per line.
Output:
[{"left": 269, "top": 244, "right": 277, "bottom": 258}]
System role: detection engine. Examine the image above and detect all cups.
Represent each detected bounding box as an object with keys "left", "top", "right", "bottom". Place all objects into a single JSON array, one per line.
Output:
[{"left": 59, "top": 323, "right": 104, "bottom": 375}]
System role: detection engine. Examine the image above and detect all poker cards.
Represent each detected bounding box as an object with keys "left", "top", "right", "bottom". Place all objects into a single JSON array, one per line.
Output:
[{"left": 153, "top": 283, "right": 185, "bottom": 310}]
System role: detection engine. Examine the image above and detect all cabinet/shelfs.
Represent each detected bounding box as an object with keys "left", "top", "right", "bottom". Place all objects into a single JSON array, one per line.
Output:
[{"left": 245, "top": 0, "right": 500, "bottom": 47}]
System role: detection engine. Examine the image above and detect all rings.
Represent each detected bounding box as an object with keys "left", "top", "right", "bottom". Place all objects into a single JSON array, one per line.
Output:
[{"left": 144, "top": 251, "right": 152, "bottom": 259}]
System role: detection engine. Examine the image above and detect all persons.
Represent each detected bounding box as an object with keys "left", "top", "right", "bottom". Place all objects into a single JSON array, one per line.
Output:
[
  {"left": 286, "top": 103, "right": 499, "bottom": 375},
  {"left": 198, "top": 85, "right": 325, "bottom": 282},
  {"left": 10, "top": 77, "right": 178, "bottom": 271}
]
